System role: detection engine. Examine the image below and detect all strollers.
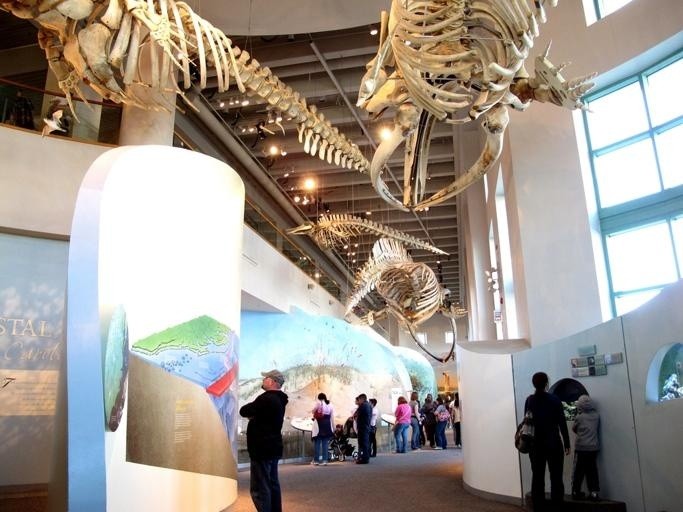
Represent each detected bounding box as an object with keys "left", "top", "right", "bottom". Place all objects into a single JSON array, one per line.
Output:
[{"left": 329, "top": 416, "right": 358, "bottom": 462}]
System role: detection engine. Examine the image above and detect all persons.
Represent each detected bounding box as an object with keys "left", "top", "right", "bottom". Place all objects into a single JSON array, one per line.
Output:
[
  {"left": 239, "top": 370, "right": 288, "bottom": 512},
  {"left": 514, "top": 372, "right": 571, "bottom": 512},
  {"left": 572, "top": 394, "right": 600, "bottom": 502},
  {"left": 310, "top": 392, "right": 461, "bottom": 466}
]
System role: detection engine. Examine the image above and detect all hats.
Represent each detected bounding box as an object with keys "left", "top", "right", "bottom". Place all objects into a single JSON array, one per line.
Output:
[{"left": 261, "top": 369, "right": 286, "bottom": 385}]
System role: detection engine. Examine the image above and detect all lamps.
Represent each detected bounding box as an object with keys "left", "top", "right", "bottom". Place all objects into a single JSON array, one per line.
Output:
[
  {"left": 216, "top": 95, "right": 292, "bottom": 132},
  {"left": 485, "top": 268, "right": 499, "bottom": 292}
]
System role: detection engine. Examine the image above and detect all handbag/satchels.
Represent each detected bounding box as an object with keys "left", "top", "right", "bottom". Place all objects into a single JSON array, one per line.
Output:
[
  {"left": 438, "top": 410, "right": 451, "bottom": 422},
  {"left": 515, "top": 405, "right": 535, "bottom": 455}
]
